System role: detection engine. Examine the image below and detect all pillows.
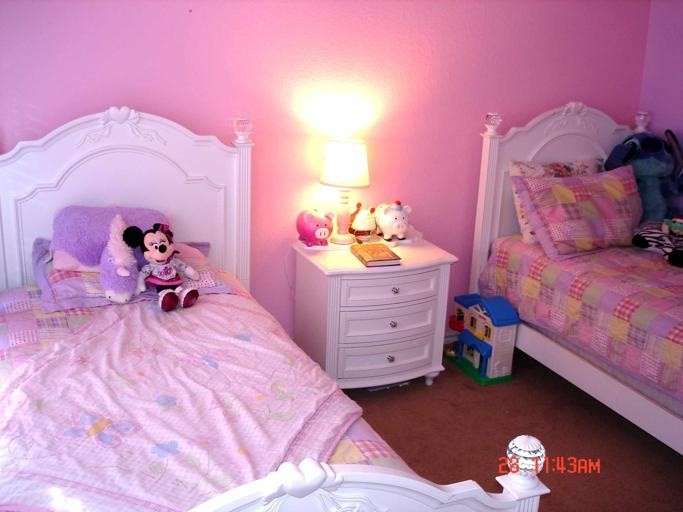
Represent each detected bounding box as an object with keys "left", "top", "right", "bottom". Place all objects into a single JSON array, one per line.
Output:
[
  {"left": 511, "top": 164, "right": 644, "bottom": 259},
  {"left": 50, "top": 205, "right": 174, "bottom": 266},
  {"left": 30, "top": 238, "right": 235, "bottom": 313},
  {"left": 509, "top": 157, "right": 607, "bottom": 245}
]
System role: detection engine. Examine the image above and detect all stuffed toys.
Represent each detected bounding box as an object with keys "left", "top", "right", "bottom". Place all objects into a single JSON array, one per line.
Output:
[
  {"left": 123, "top": 226, "right": 200, "bottom": 312},
  {"left": 99, "top": 214, "right": 139, "bottom": 303},
  {"left": 605, "top": 131, "right": 683, "bottom": 222},
  {"left": 663, "top": 215, "right": 683, "bottom": 268}
]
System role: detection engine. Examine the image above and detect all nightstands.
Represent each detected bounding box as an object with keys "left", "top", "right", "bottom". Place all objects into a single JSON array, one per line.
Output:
[{"left": 290, "top": 236, "right": 459, "bottom": 390}]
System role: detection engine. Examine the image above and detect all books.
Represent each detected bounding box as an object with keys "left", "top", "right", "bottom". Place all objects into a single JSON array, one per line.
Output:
[{"left": 350, "top": 242, "right": 402, "bottom": 267}]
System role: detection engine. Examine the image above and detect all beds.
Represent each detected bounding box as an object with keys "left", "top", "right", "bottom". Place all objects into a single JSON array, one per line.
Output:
[
  {"left": 469, "top": 100, "right": 683, "bottom": 464},
  {"left": 1, "top": 107, "right": 552, "bottom": 511}
]
